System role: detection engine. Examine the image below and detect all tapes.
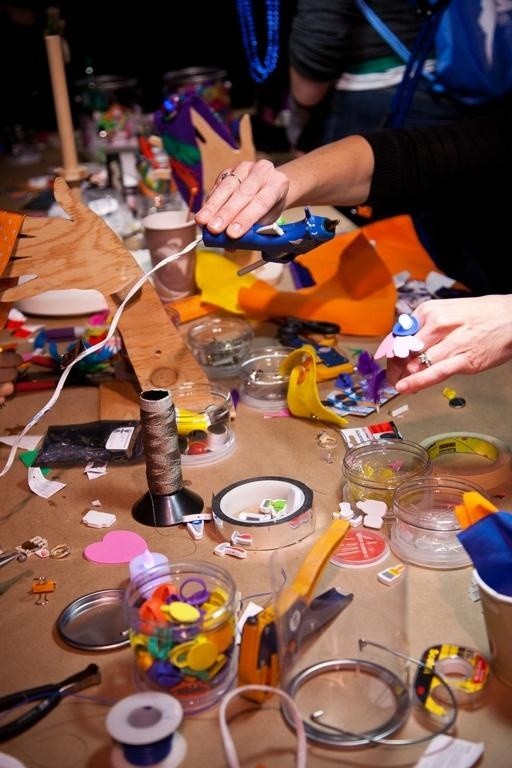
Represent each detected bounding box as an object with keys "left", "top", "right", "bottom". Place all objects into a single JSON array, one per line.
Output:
[{"left": 211, "top": 477, "right": 314, "bottom": 551}]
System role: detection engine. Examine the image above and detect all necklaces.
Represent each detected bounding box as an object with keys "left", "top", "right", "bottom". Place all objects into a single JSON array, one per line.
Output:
[{"left": 234, "top": 0, "right": 281, "bottom": 83}]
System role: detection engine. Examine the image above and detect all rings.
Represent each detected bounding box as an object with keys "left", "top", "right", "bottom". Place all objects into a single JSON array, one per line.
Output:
[
  {"left": 416, "top": 350, "right": 434, "bottom": 367},
  {"left": 220, "top": 171, "right": 242, "bottom": 185}
]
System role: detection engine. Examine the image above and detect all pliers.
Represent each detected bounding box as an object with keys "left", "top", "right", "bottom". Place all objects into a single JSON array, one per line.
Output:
[
  {"left": 14, "top": 355, "right": 99, "bottom": 392},
  {"left": 0, "top": 664, "right": 101, "bottom": 744}
]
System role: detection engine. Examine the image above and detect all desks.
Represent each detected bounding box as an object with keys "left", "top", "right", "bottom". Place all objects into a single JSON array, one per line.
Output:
[{"left": 0, "top": 124, "right": 512, "bottom": 768}]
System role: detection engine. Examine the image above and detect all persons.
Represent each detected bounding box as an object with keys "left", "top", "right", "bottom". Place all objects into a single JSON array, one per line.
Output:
[
  {"left": 286, "top": 0, "right": 463, "bottom": 146},
  {"left": 0, "top": 174, "right": 223, "bottom": 420},
  {"left": 0, "top": 350, "right": 23, "bottom": 407},
  {"left": 0, "top": 1, "right": 89, "bottom": 146},
  {"left": 195, "top": 88, "right": 512, "bottom": 395},
  {"left": 223, "top": 0, "right": 293, "bottom": 123}
]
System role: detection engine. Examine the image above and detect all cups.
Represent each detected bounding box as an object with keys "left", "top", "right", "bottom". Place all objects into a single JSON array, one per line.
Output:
[{"left": 141, "top": 206, "right": 200, "bottom": 305}]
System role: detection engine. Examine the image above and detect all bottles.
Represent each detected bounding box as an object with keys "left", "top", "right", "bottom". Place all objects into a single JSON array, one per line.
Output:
[{"left": 122, "top": 558, "right": 241, "bottom": 717}]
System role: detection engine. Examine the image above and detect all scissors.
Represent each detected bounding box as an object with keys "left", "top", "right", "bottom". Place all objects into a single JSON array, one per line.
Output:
[{"left": 278, "top": 314, "right": 340, "bottom": 335}]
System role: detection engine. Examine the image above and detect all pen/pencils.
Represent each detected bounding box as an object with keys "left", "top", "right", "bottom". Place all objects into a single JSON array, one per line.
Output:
[{"left": 137, "top": 133, "right": 161, "bottom": 169}]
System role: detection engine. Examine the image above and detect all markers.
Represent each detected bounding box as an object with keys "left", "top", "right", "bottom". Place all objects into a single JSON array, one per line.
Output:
[{"left": 45, "top": 327, "right": 88, "bottom": 340}]
